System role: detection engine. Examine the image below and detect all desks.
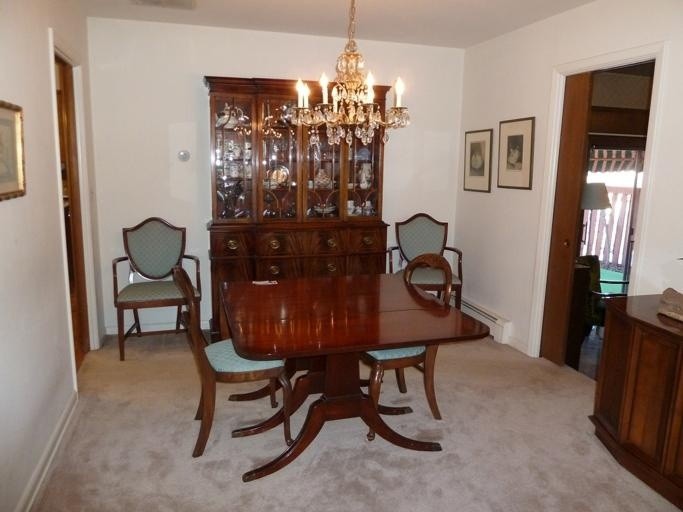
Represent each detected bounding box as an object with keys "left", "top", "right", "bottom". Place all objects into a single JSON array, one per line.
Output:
[
  {"left": 220, "top": 273, "right": 490, "bottom": 483},
  {"left": 565, "top": 263, "right": 590, "bottom": 363}
]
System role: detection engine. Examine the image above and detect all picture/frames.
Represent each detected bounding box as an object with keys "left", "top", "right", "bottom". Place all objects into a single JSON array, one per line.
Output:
[
  {"left": 463, "top": 129, "right": 492, "bottom": 193},
  {"left": 498, "top": 117, "right": 536, "bottom": 190},
  {"left": 0, "top": 101, "right": 26, "bottom": 201}
]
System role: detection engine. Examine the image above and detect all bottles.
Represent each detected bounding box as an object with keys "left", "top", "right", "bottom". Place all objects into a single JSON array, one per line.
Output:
[{"left": 312, "top": 168, "right": 330, "bottom": 189}]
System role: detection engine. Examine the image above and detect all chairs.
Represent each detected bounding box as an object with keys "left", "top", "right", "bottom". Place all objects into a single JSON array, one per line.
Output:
[
  {"left": 576, "top": 255, "right": 629, "bottom": 336},
  {"left": 359, "top": 253, "right": 453, "bottom": 421},
  {"left": 112, "top": 217, "right": 202, "bottom": 360},
  {"left": 388, "top": 212, "right": 462, "bottom": 310},
  {"left": 173, "top": 265, "right": 294, "bottom": 458}
]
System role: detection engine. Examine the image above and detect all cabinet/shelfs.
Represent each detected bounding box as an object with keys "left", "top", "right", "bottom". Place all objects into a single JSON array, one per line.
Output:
[
  {"left": 208, "top": 80, "right": 303, "bottom": 220},
  {"left": 303, "top": 77, "right": 383, "bottom": 219},
  {"left": 213, "top": 225, "right": 387, "bottom": 255},
  {"left": 588, "top": 293, "right": 683, "bottom": 512},
  {"left": 214, "top": 258, "right": 300, "bottom": 276},
  {"left": 302, "top": 256, "right": 384, "bottom": 274}
]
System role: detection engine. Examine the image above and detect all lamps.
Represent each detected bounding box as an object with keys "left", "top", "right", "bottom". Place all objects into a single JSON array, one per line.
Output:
[
  {"left": 581, "top": 182, "right": 612, "bottom": 255},
  {"left": 290, "top": 0, "right": 409, "bottom": 145}
]
